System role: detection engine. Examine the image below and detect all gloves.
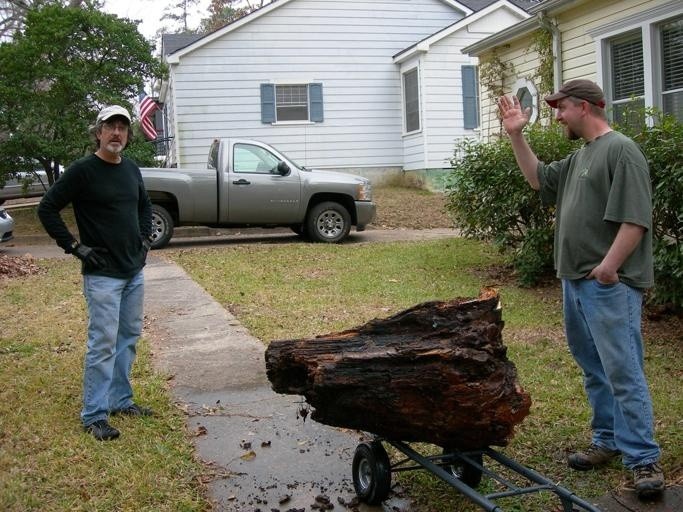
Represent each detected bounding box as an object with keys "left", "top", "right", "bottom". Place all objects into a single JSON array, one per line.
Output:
[{"left": 71, "top": 242, "right": 110, "bottom": 272}]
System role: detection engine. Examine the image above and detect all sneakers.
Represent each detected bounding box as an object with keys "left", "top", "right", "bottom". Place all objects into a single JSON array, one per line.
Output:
[
  {"left": 83, "top": 417, "right": 120, "bottom": 441},
  {"left": 123, "top": 400, "right": 160, "bottom": 421},
  {"left": 565, "top": 441, "right": 623, "bottom": 471},
  {"left": 632, "top": 462, "right": 667, "bottom": 499}
]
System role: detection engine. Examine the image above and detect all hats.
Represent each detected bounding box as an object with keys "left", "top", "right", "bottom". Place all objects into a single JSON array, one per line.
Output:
[
  {"left": 95, "top": 104, "right": 134, "bottom": 130},
  {"left": 542, "top": 78, "right": 607, "bottom": 110}
]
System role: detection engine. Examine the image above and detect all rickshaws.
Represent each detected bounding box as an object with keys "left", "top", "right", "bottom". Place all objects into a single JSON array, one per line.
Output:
[{"left": 350, "top": 423, "right": 604, "bottom": 512}]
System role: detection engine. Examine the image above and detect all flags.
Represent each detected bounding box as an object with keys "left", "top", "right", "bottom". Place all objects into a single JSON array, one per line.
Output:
[{"left": 136, "top": 85, "right": 159, "bottom": 143}]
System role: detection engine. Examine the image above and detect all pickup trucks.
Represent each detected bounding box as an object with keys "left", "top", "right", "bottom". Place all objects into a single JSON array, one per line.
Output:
[{"left": 133, "top": 136, "right": 377, "bottom": 251}]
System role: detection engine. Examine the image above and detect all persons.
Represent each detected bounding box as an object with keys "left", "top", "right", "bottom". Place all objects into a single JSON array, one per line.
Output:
[
  {"left": 37, "top": 102, "right": 157, "bottom": 441},
  {"left": 495, "top": 78, "right": 667, "bottom": 502}
]
